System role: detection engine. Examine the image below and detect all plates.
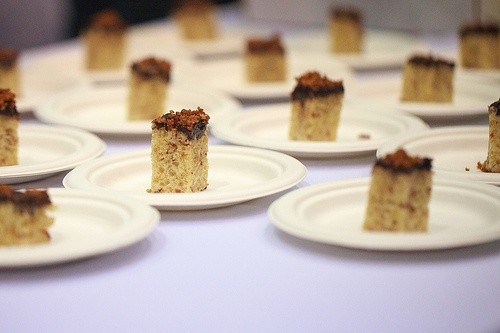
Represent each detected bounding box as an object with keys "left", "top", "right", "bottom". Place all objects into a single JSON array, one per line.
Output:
[{"left": 0, "top": 28, "right": 500, "bottom": 270}]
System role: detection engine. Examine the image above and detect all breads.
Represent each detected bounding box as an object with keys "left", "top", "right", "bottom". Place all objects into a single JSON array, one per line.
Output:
[
  {"left": 0, "top": 89, "right": 21, "bottom": 167},
  {"left": 477, "top": 98, "right": 500, "bottom": 173},
  {"left": 363, "top": 149, "right": 432, "bottom": 231},
  {"left": 456, "top": 22, "right": 500, "bottom": 69},
  {"left": 0, "top": 44, "right": 27, "bottom": 95},
  {"left": 329, "top": 7, "right": 362, "bottom": 52},
  {"left": 81, "top": 11, "right": 124, "bottom": 70},
  {"left": 172, "top": 1, "right": 217, "bottom": 41},
  {"left": 400, "top": 53, "right": 455, "bottom": 104},
  {"left": 289, "top": 71, "right": 344, "bottom": 142},
  {"left": 0, "top": 183, "right": 56, "bottom": 248},
  {"left": 129, "top": 57, "right": 170, "bottom": 120},
  {"left": 147, "top": 106, "right": 210, "bottom": 194},
  {"left": 243, "top": 32, "right": 287, "bottom": 83}
]
